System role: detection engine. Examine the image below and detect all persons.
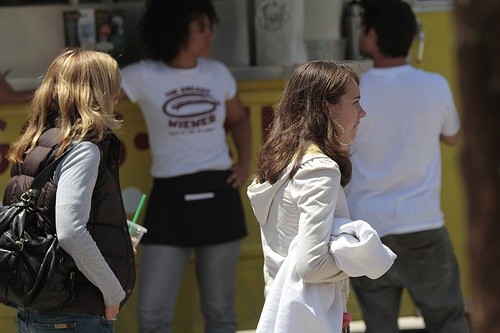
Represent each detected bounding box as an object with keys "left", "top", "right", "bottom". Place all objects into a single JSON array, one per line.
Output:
[
  {"left": 115, "top": 0, "right": 251, "bottom": 333},
  {"left": 343, "top": 0, "right": 470, "bottom": 333},
  {"left": 246, "top": 60, "right": 367, "bottom": 333},
  {"left": 0, "top": 49, "right": 137, "bottom": 333}
]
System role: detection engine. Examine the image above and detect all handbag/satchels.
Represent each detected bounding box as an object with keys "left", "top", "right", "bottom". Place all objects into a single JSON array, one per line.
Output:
[{"left": 0, "top": 140, "right": 102, "bottom": 312}]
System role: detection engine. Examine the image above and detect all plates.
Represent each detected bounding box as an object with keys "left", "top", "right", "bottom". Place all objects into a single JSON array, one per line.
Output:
[
  {"left": 5, "top": 77, "right": 41, "bottom": 92},
  {"left": 229, "top": 66, "right": 283, "bottom": 80}
]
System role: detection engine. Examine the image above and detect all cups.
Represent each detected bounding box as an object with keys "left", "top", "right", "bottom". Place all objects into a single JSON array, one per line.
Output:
[{"left": 126, "top": 219, "right": 148, "bottom": 250}]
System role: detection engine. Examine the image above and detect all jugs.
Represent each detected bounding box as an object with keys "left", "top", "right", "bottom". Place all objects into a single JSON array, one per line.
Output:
[{"left": 339, "top": 0, "right": 365, "bottom": 58}]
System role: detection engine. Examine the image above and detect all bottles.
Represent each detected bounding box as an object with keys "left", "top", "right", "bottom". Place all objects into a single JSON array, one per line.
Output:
[
  {"left": 62, "top": 9, "right": 125, "bottom": 51},
  {"left": 342, "top": 313, "right": 351, "bottom": 333}
]
